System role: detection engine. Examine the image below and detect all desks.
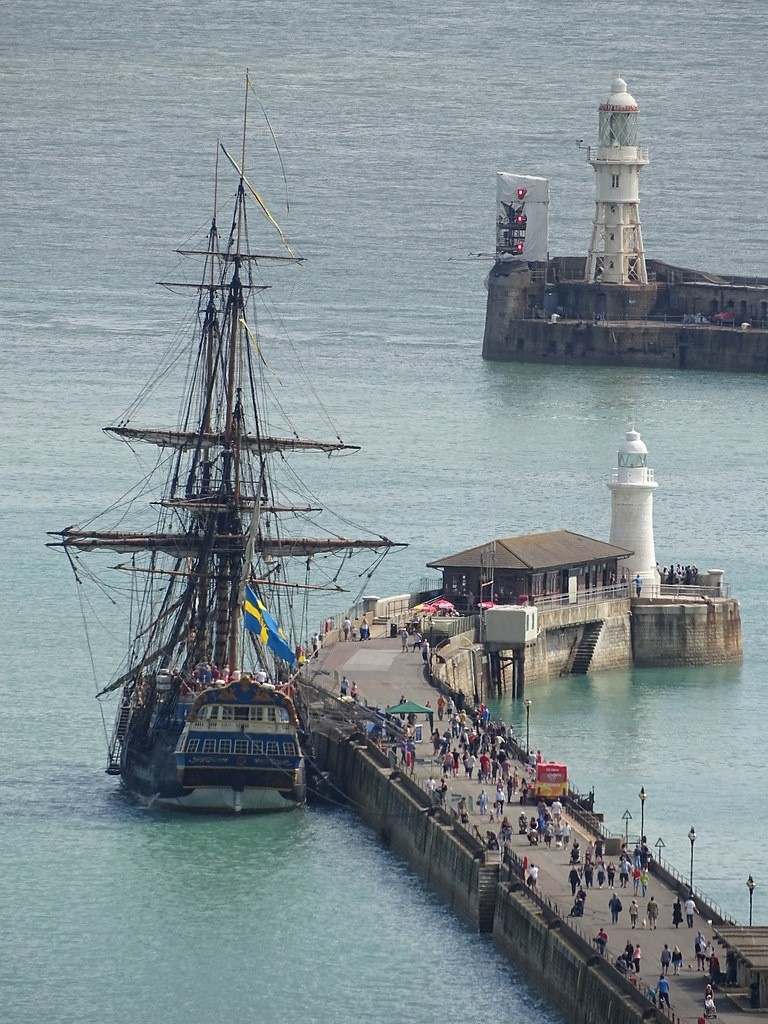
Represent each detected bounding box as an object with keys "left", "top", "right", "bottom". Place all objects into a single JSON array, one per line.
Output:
[{"left": 405, "top": 622, "right": 418, "bottom": 635}]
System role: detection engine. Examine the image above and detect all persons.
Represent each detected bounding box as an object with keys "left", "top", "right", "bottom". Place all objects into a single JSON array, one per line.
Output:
[
  {"left": 610, "top": 562, "right": 699, "bottom": 598},
  {"left": 566, "top": 833, "right": 733, "bottom": 1018},
  {"left": 464, "top": 588, "right": 476, "bottom": 611},
  {"left": 312, "top": 608, "right": 372, "bottom": 659},
  {"left": 386, "top": 688, "right": 571, "bottom": 891},
  {"left": 340, "top": 676, "right": 359, "bottom": 701},
  {"left": 400, "top": 627, "right": 430, "bottom": 666},
  {"left": 137, "top": 663, "right": 267, "bottom": 703}
]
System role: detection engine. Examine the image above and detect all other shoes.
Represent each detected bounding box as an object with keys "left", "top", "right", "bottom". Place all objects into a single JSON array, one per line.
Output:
[
  {"left": 697, "top": 967, "right": 705, "bottom": 971},
  {"left": 612, "top": 886, "right": 614, "bottom": 890},
  {"left": 624, "top": 884, "right": 626, "bottom": 888},
  {"left": 632, "top": 926, "right": 636, "bottom": 929},
  {"left": 608, "top": 886, "right": 611, "bottom": 889},
  {"left": 674, "top": 973, "right": 680, "bottom": 976},
  {"left": 643, "top": 867, "right": 648, "bottom": 872},
  {"left": 600, "top": 886, "right": 602, "bottom": 889},
  {"left": 601, "top": 884, "right": 603, "bottom": 886},
  {"left": 650, "top": 928, "right": 652, "bottom": 930},
  {"left": 654, "top": 926, "right": 656, "bottom": 929},
  {"left": 621, "top": 884, "right": 623, "bottom": 887},
  {"left": 660, "top": 972, "right": 668, "bottom": 976}
]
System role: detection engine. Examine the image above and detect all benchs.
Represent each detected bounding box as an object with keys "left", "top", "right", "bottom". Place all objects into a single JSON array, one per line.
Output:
[{"left": 398, "top": 626, "right": 419, "bottom": 635}]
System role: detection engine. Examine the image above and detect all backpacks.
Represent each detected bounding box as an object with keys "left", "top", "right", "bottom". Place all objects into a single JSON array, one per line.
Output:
[{"left": 616, "top": 901, "right": 622, "bottom": 912}]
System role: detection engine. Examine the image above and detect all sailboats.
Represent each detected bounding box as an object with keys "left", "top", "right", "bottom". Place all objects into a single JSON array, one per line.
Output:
[{"left": 45, "top": 66, "right": 411, "bottom": 812}]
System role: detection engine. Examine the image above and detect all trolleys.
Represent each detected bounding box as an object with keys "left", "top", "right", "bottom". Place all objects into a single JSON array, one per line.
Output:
[{"left": 704, "top": 1001, "right": 718, "bottom": 1020}]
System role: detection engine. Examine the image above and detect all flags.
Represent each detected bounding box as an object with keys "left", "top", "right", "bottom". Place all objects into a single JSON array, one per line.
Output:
[{"left": 244, "top": 584, "right": 296, "bottom": 663}]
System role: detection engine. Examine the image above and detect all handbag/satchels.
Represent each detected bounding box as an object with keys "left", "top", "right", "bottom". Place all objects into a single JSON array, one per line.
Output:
[
  {"left": 493, "top": 801, "right": 497, "bottom": 808},
  {"left": 476, "top": 801, "right": 480, "bottom": 805}
]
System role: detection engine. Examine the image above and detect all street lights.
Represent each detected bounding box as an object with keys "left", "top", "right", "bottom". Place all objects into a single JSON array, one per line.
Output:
[
  {"left": 520, "top": 698, "right": 532, "bottom": 755},
  {"left": 639, "top": 788, "right": 648, "bottom": 842},
  {"left": 745, "top": 874, "right": 756, "bottom": 926},
  {"left": 428, "top": 620, "right": 434, "bottom": 650},
  {"left": 688, "top": 825, "right": 696, "bottom": 894}
]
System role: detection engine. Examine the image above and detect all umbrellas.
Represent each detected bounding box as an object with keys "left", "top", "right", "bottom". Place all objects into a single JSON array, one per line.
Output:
[
  {"left": 431, "top": 599, "right": 454, "bottom": 609},
  {"left": 410, "top": 603, "right": 437, "bottom": 612},
  {"left": 478, "top": 601, "right": 497, "bottom": 609},
  {"left": 387, "top": 701, "right": 434, "bottom": 714}
]
